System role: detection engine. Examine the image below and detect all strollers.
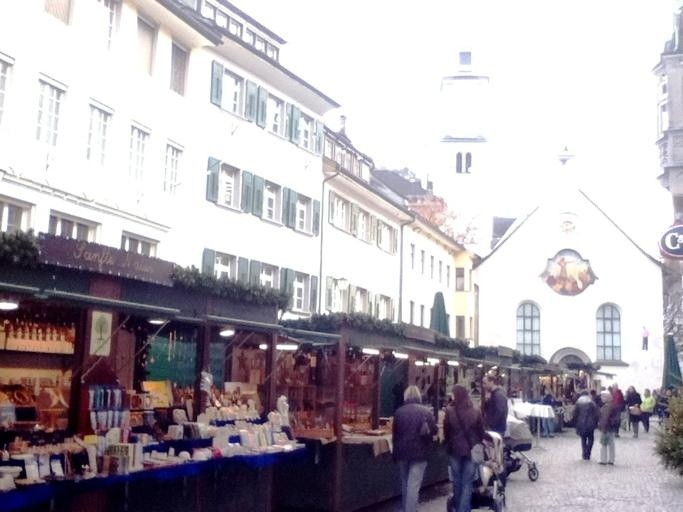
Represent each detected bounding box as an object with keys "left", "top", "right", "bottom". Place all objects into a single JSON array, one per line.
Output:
[
  {"left": 503, "top": 415, "right": 538, "bottom": 482},
  {"left": 443, "top": 430, "right": 508, "bottom": 512}
]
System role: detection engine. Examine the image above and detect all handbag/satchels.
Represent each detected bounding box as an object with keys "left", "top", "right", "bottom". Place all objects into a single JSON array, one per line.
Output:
[{"left": 629, "top": 405, "right": 642, "bottom": 417}]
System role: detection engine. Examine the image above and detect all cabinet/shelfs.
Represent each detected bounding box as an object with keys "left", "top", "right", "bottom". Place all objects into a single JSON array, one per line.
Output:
[{"left": 0, "top": 334, "right": 578, "bottom": 512}]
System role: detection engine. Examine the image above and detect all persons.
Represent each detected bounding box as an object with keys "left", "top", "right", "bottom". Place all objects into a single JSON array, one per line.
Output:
[
  {"left": 570, "top": 390, "right": 597, "bottom": 460},
  {"left": 481, "top": 374, "right": 508, "bottom": 436},
  {"left": 597, "top": 390, "right": 618, "bottom": 465},
  {"left": 538, "top": 380, "right": 673, "bottom": 439},
  {"left": 390, "top": 384, "right": 439, "bottom": 512},
  {"left": 442, "top": 384, "right": 493, "bottom": 512}
]
